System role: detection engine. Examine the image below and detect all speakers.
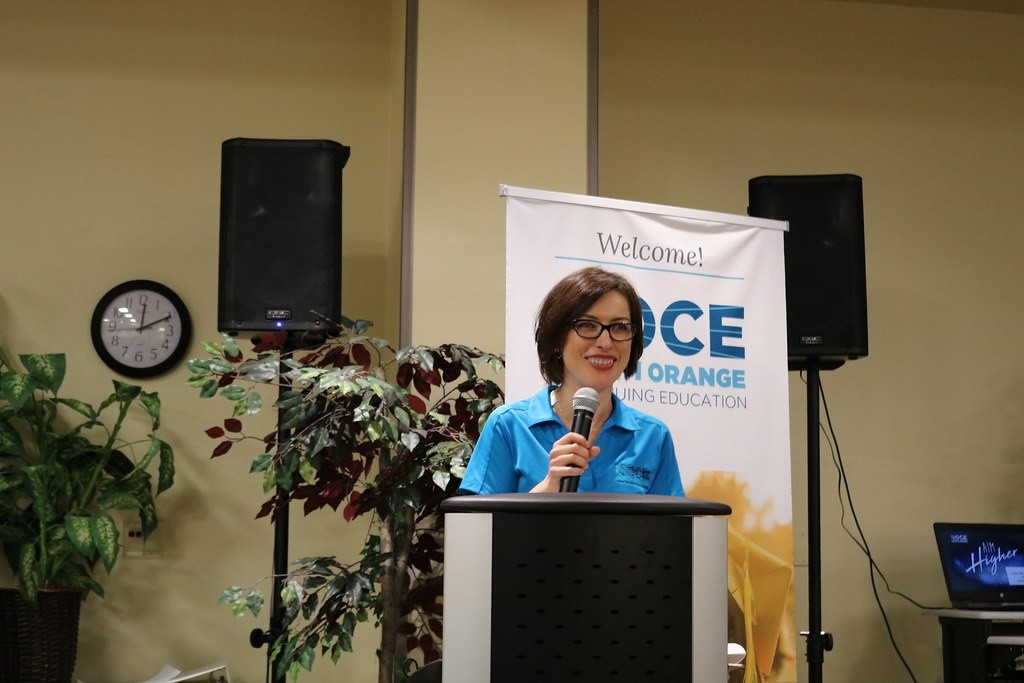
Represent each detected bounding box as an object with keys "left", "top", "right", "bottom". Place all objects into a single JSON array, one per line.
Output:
[
  {"left": 218, "top": 138, "right": 350, "bottom": 343},
  {"left": 747, "top": 174, "right": 869, "bottom": 372}
]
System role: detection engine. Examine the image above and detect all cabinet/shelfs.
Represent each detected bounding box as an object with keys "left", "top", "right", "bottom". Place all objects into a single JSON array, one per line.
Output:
[{"left": 922, "top": 609, "right": 1024, "bottom": 683}]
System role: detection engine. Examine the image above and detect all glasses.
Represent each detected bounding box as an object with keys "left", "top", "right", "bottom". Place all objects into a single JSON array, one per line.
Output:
[{"left": 570, "top": 318, "right": 637, "bottom": 342}]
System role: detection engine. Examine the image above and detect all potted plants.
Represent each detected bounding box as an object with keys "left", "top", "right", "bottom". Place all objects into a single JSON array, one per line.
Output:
[{"left": 0, "top": 352, "right": 176, "bottom": 683}]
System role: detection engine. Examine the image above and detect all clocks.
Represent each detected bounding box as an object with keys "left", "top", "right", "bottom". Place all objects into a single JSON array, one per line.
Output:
[{"left": 91, "top": 279, "right": 192, "bottom": 379}]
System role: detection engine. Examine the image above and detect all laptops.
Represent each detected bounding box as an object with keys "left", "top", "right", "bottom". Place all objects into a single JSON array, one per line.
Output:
[{"left": 932, "top": 523, "right": 1024, "bottom": 611}]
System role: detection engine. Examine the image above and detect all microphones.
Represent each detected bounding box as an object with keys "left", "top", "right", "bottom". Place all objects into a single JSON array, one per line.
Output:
[{"left": 559, "top": 387, "right": 601, "bottom": 493}]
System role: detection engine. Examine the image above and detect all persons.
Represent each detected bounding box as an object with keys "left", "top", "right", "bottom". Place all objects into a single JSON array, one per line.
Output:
[{"left": 457, "top": 267, "right": 686, "bottom": 496}]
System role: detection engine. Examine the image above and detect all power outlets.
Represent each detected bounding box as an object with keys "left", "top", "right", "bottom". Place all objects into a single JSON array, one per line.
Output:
[{"left": 122, "top": 519, "right": 164, "bottom": 559}]
